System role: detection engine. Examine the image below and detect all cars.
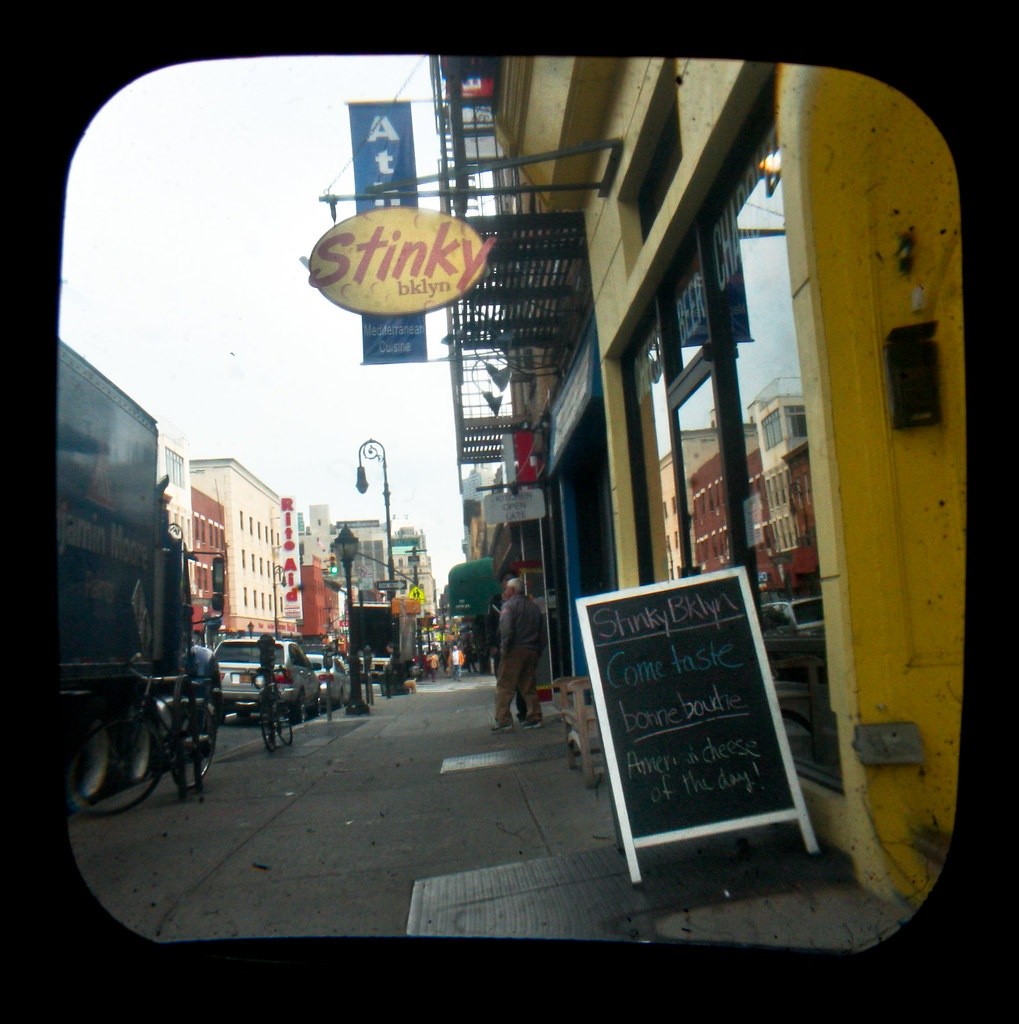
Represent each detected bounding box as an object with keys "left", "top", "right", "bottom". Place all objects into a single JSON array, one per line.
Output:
[{"left": 304, "top": 655, "right": 351, "bottom": 711}]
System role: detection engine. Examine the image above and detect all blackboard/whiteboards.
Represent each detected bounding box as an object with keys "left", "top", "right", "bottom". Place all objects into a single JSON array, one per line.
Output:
[{"left": 574, "top": 565, "right": 806, "bottom": 850}]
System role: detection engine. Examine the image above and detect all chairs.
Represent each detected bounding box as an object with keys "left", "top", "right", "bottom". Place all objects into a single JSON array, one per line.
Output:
[{"left": 554, "top": 676, "right": 607, "bottom": 789}]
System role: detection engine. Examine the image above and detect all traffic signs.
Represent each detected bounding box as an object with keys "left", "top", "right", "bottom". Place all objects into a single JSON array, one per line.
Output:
[{"left": 377, "top": 580, "right": 407, "bottom": 590}]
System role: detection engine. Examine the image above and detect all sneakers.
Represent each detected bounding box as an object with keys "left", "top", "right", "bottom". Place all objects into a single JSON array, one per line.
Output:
[
  {"left": 488, "top": 720, "right": 513, "bottom": 735},
  {"left": 520, "top": 713, "right": 543, "bottom": 729}
]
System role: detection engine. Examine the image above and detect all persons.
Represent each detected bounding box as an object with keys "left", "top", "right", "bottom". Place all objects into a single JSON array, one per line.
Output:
[
  {"left": 485, "top": 571, "right": 528, "bottom": 725},
  {"left": 491, "top": 577, "right": 543, "bottom": 732},
  {"left": 417, "top": 632, "right": 483, "bottom": 682},
  {"left": 385, "top": 645, "right": 402, "bottom": 696}
]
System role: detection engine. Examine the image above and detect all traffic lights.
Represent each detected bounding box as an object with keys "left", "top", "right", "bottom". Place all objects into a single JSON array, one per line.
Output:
[{"left": 330, "top": 556, "right": 338, "bottom": 574}]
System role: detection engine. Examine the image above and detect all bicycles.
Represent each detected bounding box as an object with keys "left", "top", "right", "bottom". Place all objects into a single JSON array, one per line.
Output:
[
  {"left": 248, "top": 666, "right": 293, "bottom": 753},
  {"left": 64, "top": 653, "right": 220, "bottom": 817}
]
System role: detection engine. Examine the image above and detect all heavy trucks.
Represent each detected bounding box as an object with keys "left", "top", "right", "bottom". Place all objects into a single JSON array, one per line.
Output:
[{"left": 51, "top": 341, "right": 226, "bottom": 824}]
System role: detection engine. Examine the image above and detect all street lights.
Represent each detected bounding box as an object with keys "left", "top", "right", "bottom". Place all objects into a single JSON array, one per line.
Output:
[
  {"left": 248, "top": 621, "right": 254, "bottom": 639},
  {"left": 355, "top": 441, "right": 411, "bottom": 696},
  {"left": 405, "top": 547, "right": 429, "bottom": 680},
  {"left": 273, "top": 565, "right": 288, "bottom": 640},
  {"left": 333, "top": 522, "right": 370, "bottom": 715}
]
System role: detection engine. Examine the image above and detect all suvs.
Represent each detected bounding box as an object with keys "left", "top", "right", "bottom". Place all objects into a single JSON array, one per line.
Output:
[{"left": 210, "top": 639, "right": 321, "bottom": 727}]
